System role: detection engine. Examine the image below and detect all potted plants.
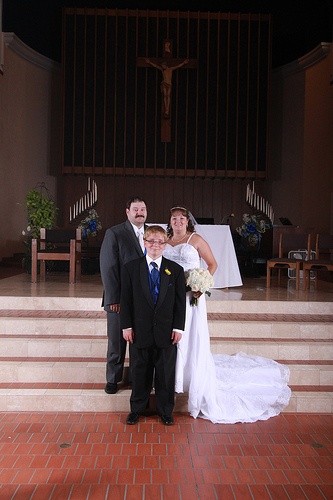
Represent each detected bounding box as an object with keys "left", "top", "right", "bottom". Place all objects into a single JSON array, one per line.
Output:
[{"left": 17, "top": 183, "right": 59, "bottom": 272}]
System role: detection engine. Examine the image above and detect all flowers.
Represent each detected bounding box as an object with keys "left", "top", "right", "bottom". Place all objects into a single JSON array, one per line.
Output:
[
  {"left": 235, "top": 213, "right": 266, "bottom": 245},
  {"left": 79, "top": 209, "right": 102, "bottom": 239},
  {"left": 187, "top": 268, "right": 214, "bottom": 306}
]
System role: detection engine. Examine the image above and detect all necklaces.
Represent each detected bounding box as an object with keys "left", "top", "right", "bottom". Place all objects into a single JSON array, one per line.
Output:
[{"left": 172, "top": 232, "right": 188, "bottom": 242}]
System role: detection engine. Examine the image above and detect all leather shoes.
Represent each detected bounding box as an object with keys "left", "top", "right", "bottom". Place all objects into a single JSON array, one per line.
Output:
[
  {"left": 160, "top": 414, "right": 173, "bottom": 425},
  {"left": 105, "top": 381, "right": 118, "bottom": 394},
  {"left": 126, "top": 411, "right": 139, "bottom": 424}
]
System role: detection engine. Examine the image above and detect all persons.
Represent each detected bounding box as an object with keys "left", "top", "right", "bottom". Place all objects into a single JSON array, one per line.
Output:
[
  {"left": 144, "top": 57, "right": 189, "bottom": 114},
  {"left": 119, "top": 226, "right": 186, "bottom": 426},
  {"left": 161, "top": 206, "right": 291, "bottom": 425},
  {"left": 99, "top": 197, "right": 149, "bottom": 394}
]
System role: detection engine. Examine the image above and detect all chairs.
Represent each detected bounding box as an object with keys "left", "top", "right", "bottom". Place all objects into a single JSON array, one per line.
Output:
[
  {"left": 267, "top": 225, "right": 305, "bottom": 291},
  {"left": 303, "top": 228, "right": 333, "bottom": 290},
  {"left": 30, "top": 228, "right": 82, "bottom": 284}
]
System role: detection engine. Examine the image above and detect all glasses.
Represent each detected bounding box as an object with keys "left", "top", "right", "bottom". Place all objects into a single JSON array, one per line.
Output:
[{"left": 144, "top": 239, "right": 167, "bottom": 245}]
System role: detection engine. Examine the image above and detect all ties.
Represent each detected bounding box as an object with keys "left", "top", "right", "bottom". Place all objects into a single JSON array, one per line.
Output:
[
  {"left": 137, "top": 229, "right": 145, "bottom": 253},
  {"left": 150, "top": 262, "right": 160, "bottom": 306}
]
